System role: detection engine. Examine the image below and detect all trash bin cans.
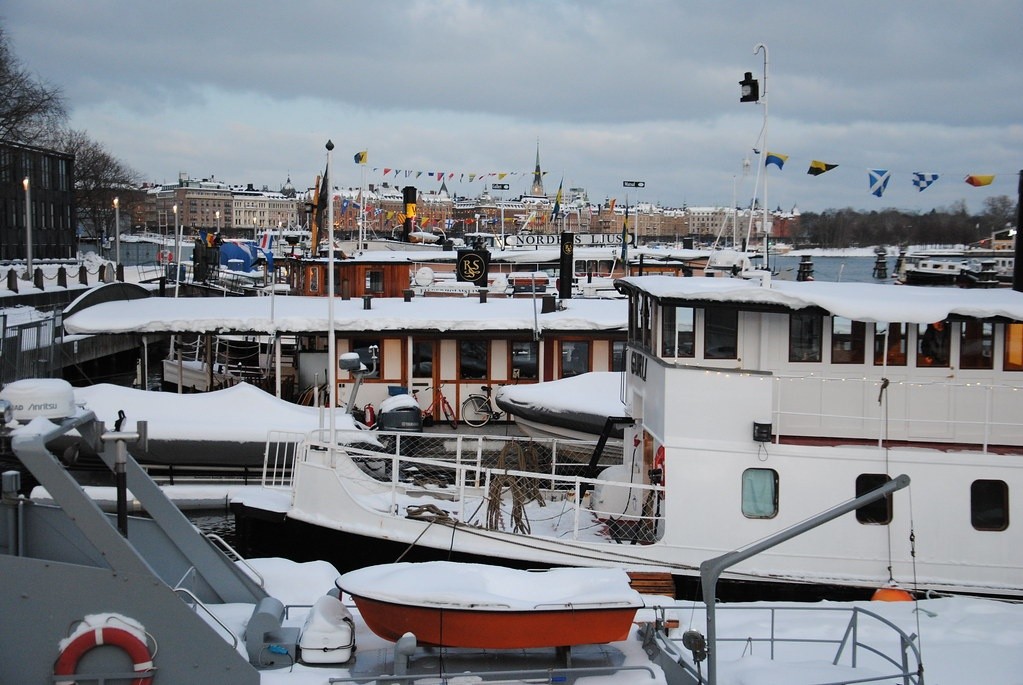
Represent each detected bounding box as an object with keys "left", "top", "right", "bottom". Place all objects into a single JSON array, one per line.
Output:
[{"left": 377, "top": 405, "right": 423, "bottom": 434}]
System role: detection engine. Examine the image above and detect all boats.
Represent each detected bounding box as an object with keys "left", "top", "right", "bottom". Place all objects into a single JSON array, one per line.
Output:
[
  {"left": 0, "top": 241, "right": 1022, "bottom": 685},
  {"left": 703, "top": 44, "right": 771, "bottom": 285},
  {"left": 896, "top": 256, "right": 1015, "bottom": 284}
]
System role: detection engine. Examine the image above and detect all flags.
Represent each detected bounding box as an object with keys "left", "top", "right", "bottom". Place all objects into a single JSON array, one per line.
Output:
[
  {"left": 621, "top": 205, "right": 630, "bottom": 271},
  {"left": 549, "top": 178, "right": 564, "bottom": 223},
  {"left": 806, "top": 159, "right": 839, "bottom": 176},
  {"left": 865, "top": 168, "right": 891, "bottom": 198},
  {"left": 353, "top": 151, "right": 367, "bottom": 164},
  {"left": 964, "top": 175, "right": 995, "bottom": 187},
  {"left": 912, "top": 172, "right": 939, "bottom": 192},
  {"left": 764, "top": 152, "right": 790, "bottom": 170},
  {"left": 196, "top": 229, "right": 216, "bottom": 247}
]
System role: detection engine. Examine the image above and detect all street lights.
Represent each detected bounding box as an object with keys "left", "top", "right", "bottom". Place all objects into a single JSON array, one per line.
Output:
[
  {"left": 215, "top": 209, "right": 221, "bottom": 234},
  {"left": 112, "top": 195, "right": 121, "bottom": 265},
  {"left": 171, "top": 201, "right": 180, "bottom": 263},
  {"left": 21, "top": 174, "right": 34, "bottom": 280}
]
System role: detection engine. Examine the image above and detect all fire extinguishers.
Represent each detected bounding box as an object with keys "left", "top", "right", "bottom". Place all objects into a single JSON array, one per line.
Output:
[{"left": 363, "top": 402, "right": 374, "bottom": 426}]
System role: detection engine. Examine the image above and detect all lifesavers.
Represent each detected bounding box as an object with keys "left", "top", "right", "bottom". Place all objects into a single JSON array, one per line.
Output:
[
  {"left": 53, "top": 626, "right": 155, "bottom": 685},
  {"left": 157, "top": 249, "right": 173, "bottom": 262}
]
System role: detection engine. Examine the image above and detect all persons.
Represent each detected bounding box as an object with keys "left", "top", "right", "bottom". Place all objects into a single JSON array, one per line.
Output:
[{"left": 919, "top": 320, "right": 964, "bottom": 365}]
